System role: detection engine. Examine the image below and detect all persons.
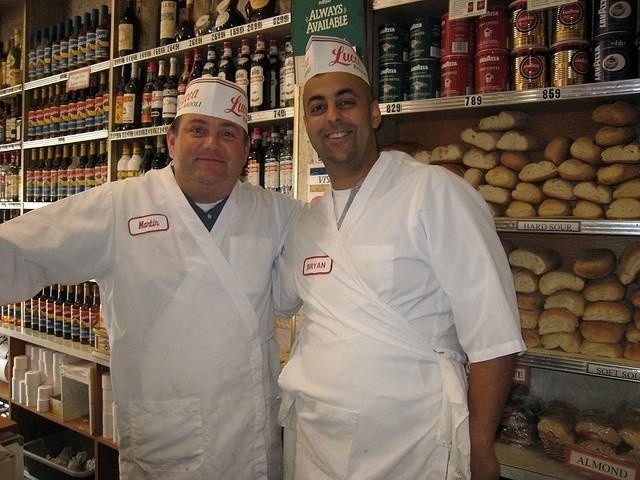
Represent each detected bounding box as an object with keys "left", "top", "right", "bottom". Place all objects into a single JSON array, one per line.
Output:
[
  {"left": 0, "top": 76, "right": 306, "bottom": 480},
  {"left": 272, "top": 35, "right": 527, "bottom": 479}
]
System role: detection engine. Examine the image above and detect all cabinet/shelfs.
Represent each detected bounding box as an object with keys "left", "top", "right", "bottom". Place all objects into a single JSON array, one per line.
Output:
[
  {"left": 0, "top": 321, "right": 119, "bottom": 479},
  {"left": 20, "top": 0, "right": 115, "bottom": 353},
  {"left": 0, "top": 1, "right": 25, "bottom": 329},
  {"left": 363, "top": 0, "right": 640, "bottom": 480},
  {"left": 107, "top": 0, "right": 295, "bottom": 363}
]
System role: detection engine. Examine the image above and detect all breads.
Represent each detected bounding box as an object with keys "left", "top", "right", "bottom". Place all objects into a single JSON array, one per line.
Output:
[
  {"left": 502, "top": 239, "right": 640, "bottom": 362},
  {"left": 495, "top": 391, "right": 640, "bottom": 463},
  {"left": 379, "top": 101, "right": 639, "bottom": 219}
]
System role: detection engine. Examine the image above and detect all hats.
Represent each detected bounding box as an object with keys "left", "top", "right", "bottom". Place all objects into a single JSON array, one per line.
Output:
[
  {"left": 303, "top": 35, "right": 371, "bottom": 86},
  {"left": 174, "top": 77, "right": 249, "bottom": 136}
]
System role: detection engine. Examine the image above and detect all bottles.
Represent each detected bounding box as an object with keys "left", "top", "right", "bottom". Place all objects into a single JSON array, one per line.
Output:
[
  {"left": 244, "top": 120, "right": 292, "bottom": 194},
  {"left": 0, "top": 5, "right": 109, "bottom": 202},
  {"left": 175, "top": 0, "right": 195, "bottom": 42},
  {"left": 117, "top": 135, "right": 171, "bottom": 178},
  {"left": 117, "top": 0, "right": 140, "bottom": 56},
  {"left": 160, "top": 0, "right": 180, "bottom": 46},
  {"left": 0, "top": 281, "right": 101, "bottom": 347},
  {"left": 113, "top": 32, "right": 294, "bottom": 131}
]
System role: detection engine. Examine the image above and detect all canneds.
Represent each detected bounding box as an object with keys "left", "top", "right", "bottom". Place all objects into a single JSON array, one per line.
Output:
[{"left": 375, "top": 0, "right": 640, "bottom": 102}]
